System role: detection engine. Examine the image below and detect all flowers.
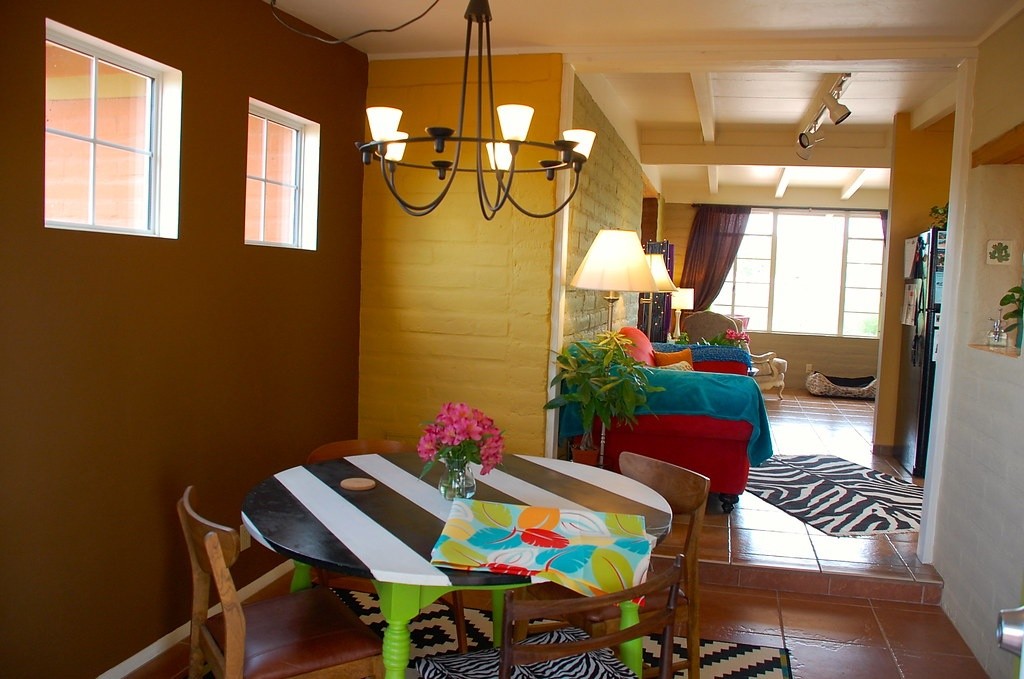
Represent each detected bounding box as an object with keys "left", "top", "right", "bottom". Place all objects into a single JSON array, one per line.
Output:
[
  {"left": 416, "top": 402, "right": 506, "bottom": 476},
  {"left": 724, "top": 329, "right": 750, "bottom": 347}
]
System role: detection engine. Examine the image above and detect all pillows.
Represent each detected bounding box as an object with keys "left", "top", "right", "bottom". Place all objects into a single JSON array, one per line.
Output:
[
  {"left": 652, "top": 347, "right": 693, "bottom": 367},
  {"left": 658, "top": 361, "right": 694, "bottom": 371}
]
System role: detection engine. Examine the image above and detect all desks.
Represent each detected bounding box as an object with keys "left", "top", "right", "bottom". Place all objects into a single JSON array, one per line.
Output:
[{"left": 241, "top": 454, "right": 673, "bottom": 679}]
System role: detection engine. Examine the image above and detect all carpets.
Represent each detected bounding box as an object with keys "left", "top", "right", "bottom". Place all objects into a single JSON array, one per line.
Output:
[
  {"left": 330, "top": 587, "right": 793, "bottom": 679},
  {"left": 744, "top": 453, "right": 925, "bottom": 537}
]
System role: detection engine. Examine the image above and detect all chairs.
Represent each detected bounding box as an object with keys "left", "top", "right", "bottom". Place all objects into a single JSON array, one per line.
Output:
[{"left": 176, "top": 437, "right": 710, "bottom": 679}]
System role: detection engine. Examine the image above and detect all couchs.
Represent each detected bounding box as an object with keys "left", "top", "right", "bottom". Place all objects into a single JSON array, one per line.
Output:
[
  {"left": 681, "top": 311, "right": 788, "bottom": 400},
  {"left": 558, "top": 327, "right": 773, "bottom": 515}
]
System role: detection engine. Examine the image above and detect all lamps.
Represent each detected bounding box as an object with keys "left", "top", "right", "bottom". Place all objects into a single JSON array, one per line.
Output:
[
  {"left": 793, "top": 142, "right": 813, "bottom": 161},
  {"left": 571, "top": 230, "right": 658, "bottom": 469},
  {"left": 822, "top": 93, "right": 851, "bottom": 125},
  {"left": 798, "top": 123, "right": 824, "bottom": 149},
  {"left": 637, "top": 255, "right": 679, "bottom": 343},
  {"left": 669, "top": 288, "right": 694, "bottom": 338},
  {"left": 354, "top": 0, "right": 597, "bottom": 218}
]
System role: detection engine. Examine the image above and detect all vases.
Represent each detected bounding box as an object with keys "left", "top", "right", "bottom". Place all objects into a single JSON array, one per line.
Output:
[{"left": 438, "top": 458, "right": 476, "bottom": 500}]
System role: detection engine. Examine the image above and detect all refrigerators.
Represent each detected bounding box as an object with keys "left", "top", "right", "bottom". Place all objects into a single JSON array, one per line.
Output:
[{"left": 891, "top": 226, "right": 948, "bottom": 477}]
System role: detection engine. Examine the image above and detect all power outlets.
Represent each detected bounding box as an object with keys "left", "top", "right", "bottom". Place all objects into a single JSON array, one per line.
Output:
[{"left": 805, "top": 364, "right": 812, "bottom": 373}]
